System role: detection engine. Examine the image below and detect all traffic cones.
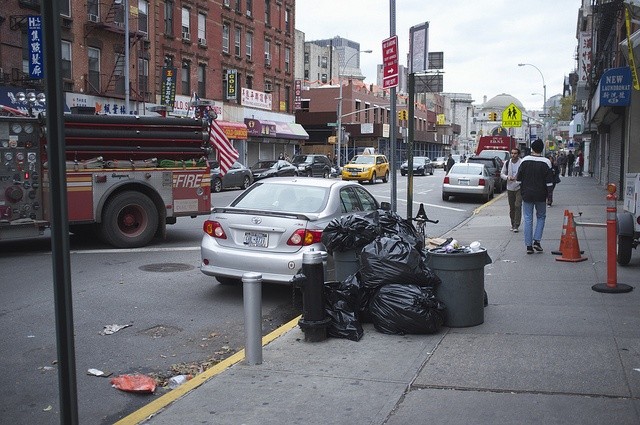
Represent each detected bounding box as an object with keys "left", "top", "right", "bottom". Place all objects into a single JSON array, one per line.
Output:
[
  {"left": 554, "top": 214, "right": 586, "bottom": 262},
  {"left": 551, "top": 210, "right": 584, "bottom": 254}
]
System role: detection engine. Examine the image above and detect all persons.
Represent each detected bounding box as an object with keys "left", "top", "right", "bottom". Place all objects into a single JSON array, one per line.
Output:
[
  {"left": 291, "top": 151, "right": 298, "bottom": 162},
  {"left": 546, "top": 151, "right": 584, "bottom": 209},
  {"left": 446, "top": 154, "right": 454, "bottom": 175},
  {"left": 500, "top": 149, "right": 521, "bottom": 233},
  {"left": 278, "top": 152, "right": 284, "bottom": 160},
  {"left": 516, "top": 139, "right": 555, "bottom": 254},
  {"left": 326, "top": 152, "right": 331, "bottom": 161}
]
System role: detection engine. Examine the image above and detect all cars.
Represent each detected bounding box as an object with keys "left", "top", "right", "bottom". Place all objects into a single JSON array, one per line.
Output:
[
  {"left": 442, "top": 163, "right": 493, "bottom": 203},
  {"left": 431, "top": 157, "right": 446, "bottom": 168},
  {"left": 200, "top": 176, "right": 390, "bottom": 284},
  {"left": 210, "top": 161, "right": 254, "bottom": 192},
  {"left": 400, "top": 156, "right": 433, "bottom": 175},
  {"left": 251, "top": 160, "right": 298, "bottom": 181}
]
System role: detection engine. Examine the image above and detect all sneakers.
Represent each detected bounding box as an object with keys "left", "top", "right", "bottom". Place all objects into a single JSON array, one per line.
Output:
[
  {"left": 534, "top": 241, "right": 543, "bottom": 251},
  {"left": 510, "top": 225, "right": 514, "bottom": 230},
  {"left": 527, "top": 245, "right": 533, "bottom": 253},
  {"left": 547, "top": 203, "right": 552, "bottom": 207},
  {"left": 514, "top": 225, "right": 518, "bottom": 232}
]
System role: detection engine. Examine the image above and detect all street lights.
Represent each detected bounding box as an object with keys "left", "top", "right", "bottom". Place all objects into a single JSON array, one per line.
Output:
[
  {"left": 517, "top": 64, "right": 545, "bottom": 157},
  {"left": 337, "top": 50, "right": 372, "bottom": 166}
]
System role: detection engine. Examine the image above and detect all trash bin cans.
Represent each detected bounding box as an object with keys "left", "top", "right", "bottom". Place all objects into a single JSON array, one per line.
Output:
[
  {"left": 332, "top": 248, "right": 360, "bottom": 281},
  {"left": 428, "top": 248, "right": 492, "bottom": 327}
]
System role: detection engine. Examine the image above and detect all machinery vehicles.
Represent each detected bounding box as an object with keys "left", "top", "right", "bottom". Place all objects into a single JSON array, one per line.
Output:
[{"left": 0, "top": 91, "right": 221, "bottom": 247}]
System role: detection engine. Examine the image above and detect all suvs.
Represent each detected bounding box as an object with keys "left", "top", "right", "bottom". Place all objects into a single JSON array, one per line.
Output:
[
  {"left": 479, "top": 149, "right": 510, "bottom": 162},
  {"left": 291, "top": 154, "right": 330, "bottom": 178},
  {"left": 467, "top": 156, "right": 506, "bottom": 193},
  {"left": 342, "top": 154, "right": 389, "bottom": 184}
]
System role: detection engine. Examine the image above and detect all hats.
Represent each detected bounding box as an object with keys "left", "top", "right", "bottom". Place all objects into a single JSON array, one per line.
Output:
[{"left": 531, "top": 138, "right": 543, "bottom": 153}]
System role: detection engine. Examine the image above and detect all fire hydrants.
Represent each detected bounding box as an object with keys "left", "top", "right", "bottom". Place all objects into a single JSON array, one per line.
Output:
[{"left": 289, "top": 248, "right": 332, "bottom": 340}]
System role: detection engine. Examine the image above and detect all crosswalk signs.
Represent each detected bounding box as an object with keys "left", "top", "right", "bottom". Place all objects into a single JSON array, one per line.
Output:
[{"left": 502, "top": 103, "right": 522, "bottom": 127}]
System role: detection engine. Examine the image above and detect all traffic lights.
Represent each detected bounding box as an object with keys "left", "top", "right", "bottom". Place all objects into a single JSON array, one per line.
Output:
[
  {"left": 488, "top": 112, "right": 497, "bottom": 121},
  {"left": 398, "top": 109, "right": 408, "bottom": 120}
]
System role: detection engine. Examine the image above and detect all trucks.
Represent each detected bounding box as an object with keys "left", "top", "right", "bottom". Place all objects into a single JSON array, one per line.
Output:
[{"left": 476, "top": 136, "right": 515, "bottom": 155}]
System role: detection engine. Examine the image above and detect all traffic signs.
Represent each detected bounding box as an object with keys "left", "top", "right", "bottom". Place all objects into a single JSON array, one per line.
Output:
[
  {"left": 328, "top": 122, "right": 338, "bottom": 127},
  {"left": 381, "top": 36, "right": 398, "bottom": 89}
]
